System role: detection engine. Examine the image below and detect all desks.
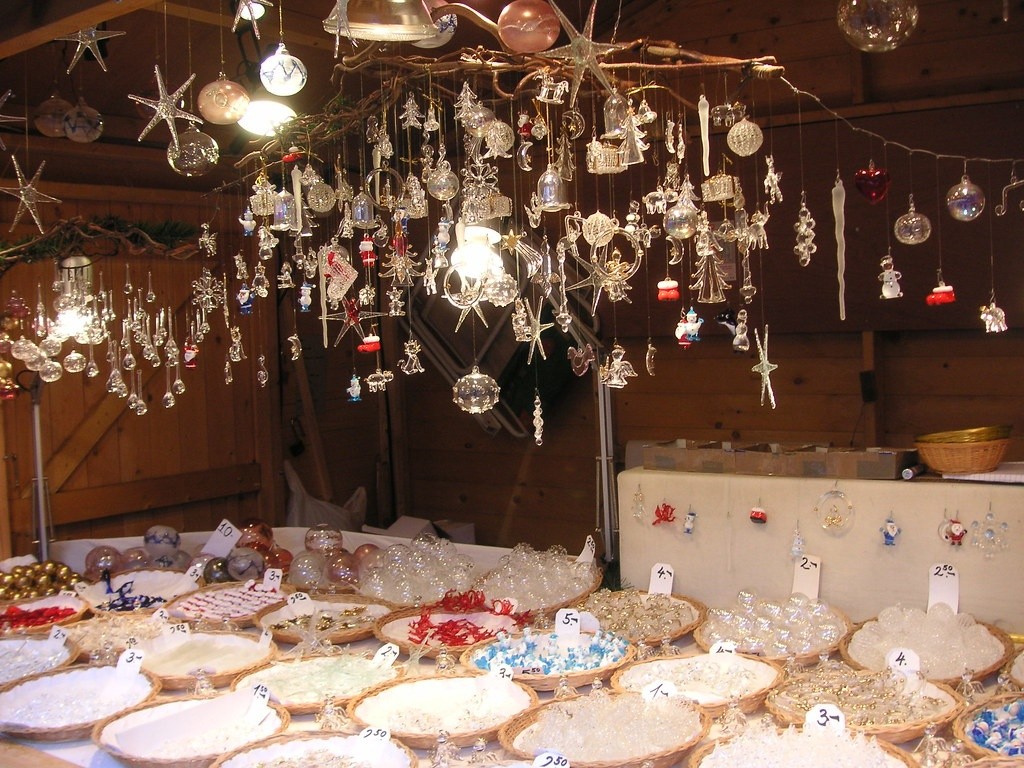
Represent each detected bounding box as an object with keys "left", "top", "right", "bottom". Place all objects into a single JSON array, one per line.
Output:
[{"left": 0, "top": 524, "right": 1023, "bottom": 767}]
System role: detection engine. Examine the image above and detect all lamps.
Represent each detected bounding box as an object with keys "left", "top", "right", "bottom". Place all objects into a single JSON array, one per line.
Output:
[
  {"left": 322, "top": 0, "right": 440, "bottom": 41},
  {"left": 236, "top": 86, "right": 298, "bottom": 137},
  {"left": 463, "top": 216, "right": 502, "bottom": 244}
]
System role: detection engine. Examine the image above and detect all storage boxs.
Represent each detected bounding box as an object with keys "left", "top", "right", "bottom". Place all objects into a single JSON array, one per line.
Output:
[{"left": 641, "top": 437, "right": 918, "bottom": 480}]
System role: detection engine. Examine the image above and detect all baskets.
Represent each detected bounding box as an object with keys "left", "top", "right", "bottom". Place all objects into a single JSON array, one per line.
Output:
[
  {"left": 913, "top": 423, "right": 1013, "bottom": 475},
  {"left": 0, "top": 560, "right": 1024, "bottom": 768}
]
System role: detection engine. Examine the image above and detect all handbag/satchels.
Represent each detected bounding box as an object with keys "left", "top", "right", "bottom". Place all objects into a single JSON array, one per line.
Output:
[
  {"left": 435, "top": 519, "right": 475, "bottom": 544},
  {"left": 282, "top": 460, "right": 366, "bottom": 531}
]
